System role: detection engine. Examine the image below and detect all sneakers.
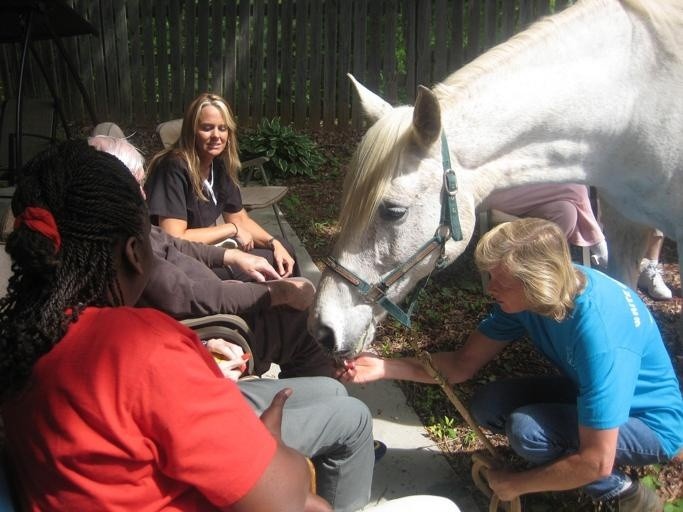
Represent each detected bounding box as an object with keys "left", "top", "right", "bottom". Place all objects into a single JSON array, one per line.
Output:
[
  {"left": 639, "top": 264, "right": 671, "bottom": 299},
  {"left": 374, "top": 441, "right": 386, "bottom": 460}
]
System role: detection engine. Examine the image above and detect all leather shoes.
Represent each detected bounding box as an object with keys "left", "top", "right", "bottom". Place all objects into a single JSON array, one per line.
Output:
[{"left": 600, "top": 475, "right": 664, "bottom": 512}]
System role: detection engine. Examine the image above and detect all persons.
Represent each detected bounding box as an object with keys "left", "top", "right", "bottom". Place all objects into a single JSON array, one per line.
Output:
[
  {"left": 145, "top": 94, "right": 302, "bottom": 280},
  {"left": 637, "top": 224, "right": 673, "bottom": 300},
  {"left": 325, "top": 218, "right": 683, "bottom": 508},
  {"left": 200, "top": 335, "right": 375, "bottom": 507},
  {"left": 86, "top": 133, "right": 387, "bottom": 460},
  {"left": 478, "top": 183, "right": 608, "bottom": 269},
  {"left": 1, "top": 140, "right": 459, "bottom": 507}
]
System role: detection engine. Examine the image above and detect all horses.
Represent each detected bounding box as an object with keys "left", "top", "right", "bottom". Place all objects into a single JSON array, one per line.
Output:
[{"left": 307, "top": 1, "right": 683, "bottom": 366}]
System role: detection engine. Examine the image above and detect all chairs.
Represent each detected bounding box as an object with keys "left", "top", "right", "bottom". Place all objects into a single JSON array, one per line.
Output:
[{"left": 157, "top": 117, "right": 290, "bottom": 240}]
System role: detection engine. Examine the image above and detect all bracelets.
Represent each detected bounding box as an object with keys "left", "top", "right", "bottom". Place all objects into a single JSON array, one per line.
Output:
[
  {"left": 230, "top": 222, "right": 238, "bottom": 239},
  {"left": 266, "top": 238, "right": 275, "bottom": 248}
]
